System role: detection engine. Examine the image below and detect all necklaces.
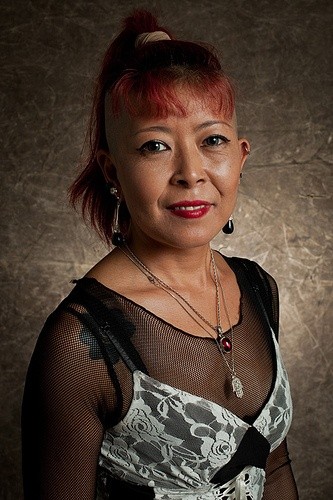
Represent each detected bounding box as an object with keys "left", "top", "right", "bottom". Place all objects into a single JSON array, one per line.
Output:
[
  {"left": 119, "top": 243, "right": 244, "bottom": 398},
  {"left": 120, "top": 233, "right": 234, "bottom": 354}
]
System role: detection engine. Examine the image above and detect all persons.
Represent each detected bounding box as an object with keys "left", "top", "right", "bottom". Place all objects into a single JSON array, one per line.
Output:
[{"left": 20, "top": 8, "right": 302, "bottom": 500}]
